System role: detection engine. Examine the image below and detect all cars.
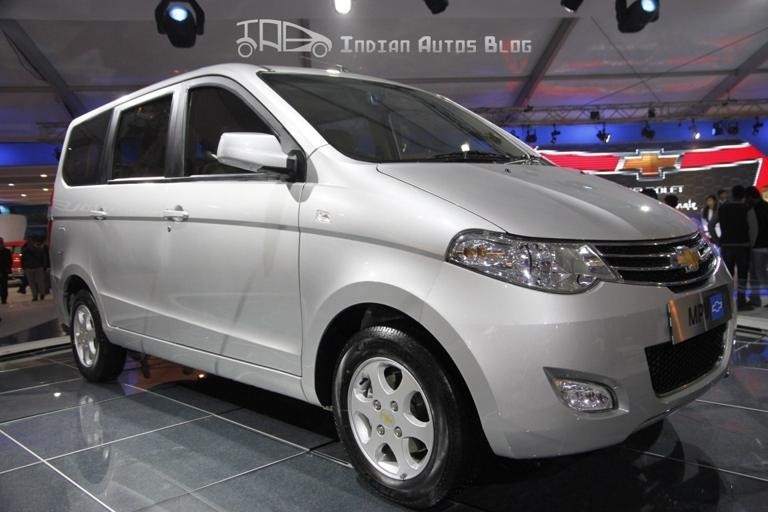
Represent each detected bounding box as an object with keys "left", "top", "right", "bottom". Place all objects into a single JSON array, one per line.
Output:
[{"left": 4, "top": 241, "right": 29, "bottom": 278}]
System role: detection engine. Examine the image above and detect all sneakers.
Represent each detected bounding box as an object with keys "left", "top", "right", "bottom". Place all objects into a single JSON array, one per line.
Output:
[
  {"left": 736, "top": 297, "right": 768, "bottom": 310},
  {"left": 17, "top": 289, "right": 44, "bottom": 301},
  {"left": 1, "top": 299, "right": 7, "bottom": 305}
]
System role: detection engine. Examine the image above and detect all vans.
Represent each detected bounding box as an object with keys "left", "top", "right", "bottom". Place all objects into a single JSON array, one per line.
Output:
[
  {"left": 49, "top": 61, "right": 740, "bottom": 512},
  {"left": 56, "top": 371, "right": 736, "bottom": 512}
]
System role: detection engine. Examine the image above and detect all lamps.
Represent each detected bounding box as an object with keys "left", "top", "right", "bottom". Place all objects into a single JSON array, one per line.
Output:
[
  {"left": 151, "top": 0, "right": 207, "bottom": 49},
  {"left": 559, "top": 0, "right": 663, "bottom": 33}
]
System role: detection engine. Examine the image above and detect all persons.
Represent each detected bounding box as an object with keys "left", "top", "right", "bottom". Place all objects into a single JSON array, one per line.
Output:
[
  {"left": 16, "top": 233, "right": 52, "bottom": 302},
  {"left": 0, "top": 237, "right": 14, "bottom": 304},
  {"left": 640, "top": 181, "right": 767, "bottom": 312}
]
